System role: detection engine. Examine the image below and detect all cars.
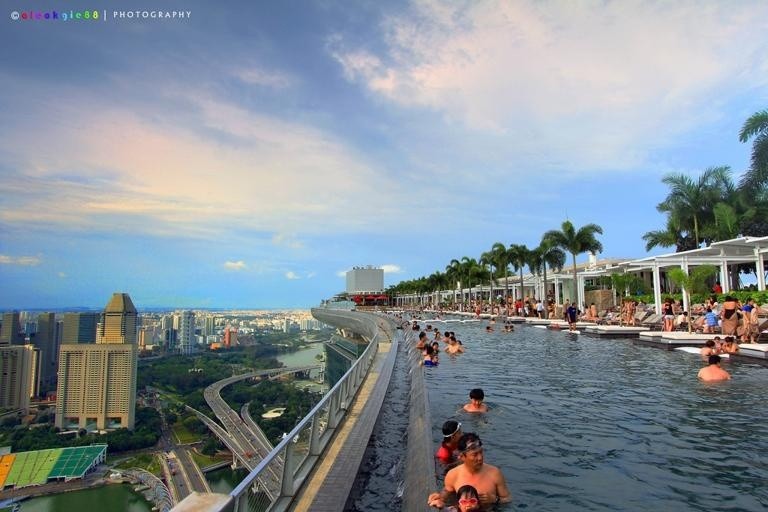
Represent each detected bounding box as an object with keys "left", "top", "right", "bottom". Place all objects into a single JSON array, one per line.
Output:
[{"left": 160, "top": 384, "right": 263, "bottom": 481}]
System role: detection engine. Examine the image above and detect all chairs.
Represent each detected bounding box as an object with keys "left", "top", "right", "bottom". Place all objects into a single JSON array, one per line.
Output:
[{"left": 582, "top": 302, "right": 767, "bottom": 342}]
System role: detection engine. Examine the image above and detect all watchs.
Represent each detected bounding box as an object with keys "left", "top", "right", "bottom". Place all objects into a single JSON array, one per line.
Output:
[{"left": 495, "top": 496, "right": 500, "bottom": 505}]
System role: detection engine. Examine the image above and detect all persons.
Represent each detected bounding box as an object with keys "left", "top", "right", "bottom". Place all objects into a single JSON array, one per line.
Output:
[
  {"left": 697, "top": 355, "right": 732, "bottom": 384},
  {"left": 462, "top": 388, "right": 488, "bottom": 414},
  {"left": 428, "top": 485, "right": 479, "bottom": 512},
  {"left": 434, "top": 420, "right": 464, "bottom": 465},
  {"left": 364, "top": 281, "right": 768, "bottom": 367},
  {"left": 426, "top": 432, "right": 511, "bottom": 509}
]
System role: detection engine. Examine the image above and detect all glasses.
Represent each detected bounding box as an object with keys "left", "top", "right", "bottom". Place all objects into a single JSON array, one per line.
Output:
[
  {"left": 467, "top": 440, "right": 482, "bottom": 451},
  {"left": 458, "top": 498, "right": 478, "bottom": 507}
]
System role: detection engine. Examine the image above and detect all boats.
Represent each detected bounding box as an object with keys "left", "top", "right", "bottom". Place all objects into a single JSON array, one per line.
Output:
[{"left": 304, "top": 384, "right": 314, "bottom": 387}]
